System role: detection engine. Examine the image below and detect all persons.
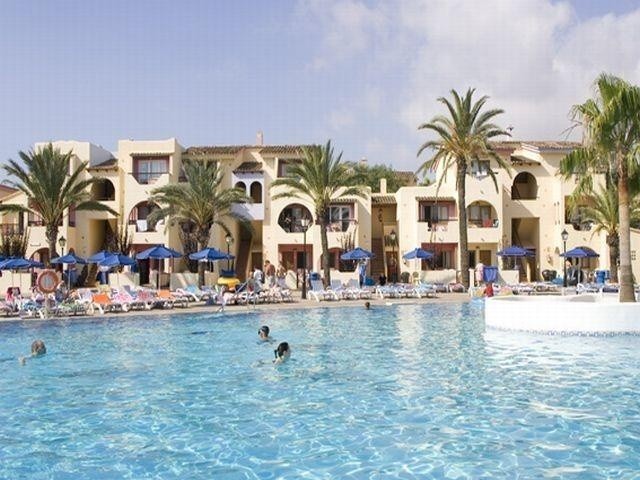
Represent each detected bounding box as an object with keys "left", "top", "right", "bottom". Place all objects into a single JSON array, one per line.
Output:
[
  {"left": 264, "top": 260, "right": 276, "bottom": 288},
  {"left": 276, "top": 261, "right": 286, "bottom": 287},
  {"left": 256, "top": 326, "right": 275, "bottom": 344},
  {"left": 274, "top": 342, "right": 292, "bottom": 365},
  {"left": 253, "top": 265, "right": 263, "bottom": 288},
  {"left": 18, "top": 340, "right": 46, "bottom": 366}
]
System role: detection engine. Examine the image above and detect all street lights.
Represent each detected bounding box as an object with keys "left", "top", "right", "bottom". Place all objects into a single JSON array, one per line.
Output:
[
  {"left": 224, "top": 233, "right": 234, "bottom": 272},
  {"left": 561, "top": 228, "right": 570, "bottom": 289},
  {"left": 58, "top": 236, "right": 66, "bottom": 279},
  {"left": 390, "top": 230, "right": 397, "bottom": 285},
  {"left": 300, "top": 214, "right": 312, "bottom": 301}
]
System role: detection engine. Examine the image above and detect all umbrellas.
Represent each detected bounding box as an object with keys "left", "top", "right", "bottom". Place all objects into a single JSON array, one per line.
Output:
[
  {"left": 496, "top": 246, "right": 536, "bottom": 269},
  {"left": 340, "top": 247, "right": 376, "bottom": 259},
  {"left": 98, "top": 253, "right": 136, "bottom": 284},
  {"left": 50, "top": 252, "right": 85, "bottom": 289},
  {"left": 86, "top": 250, "right": 111, "bottom": 263},
  {"left": 136, "top": 246, "right": 183, "bottom": 290},
  {"left": 559, "top": 246, "right": 600, "bottom": 283},
  {"left": 189, "top": 248, "right": 235, "bottom": 272},
  {"left": 402, "top": 248, "right": 433, "bottom": 270},
  {"left": 0, "top": 257, "right": 44, "bottom": 293}
]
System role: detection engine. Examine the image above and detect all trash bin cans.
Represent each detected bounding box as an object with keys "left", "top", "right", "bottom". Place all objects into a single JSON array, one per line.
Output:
[
  {"left": 311, "top": 273, "right": 317, "bottom": 280},
  {"left": 219, "top": 270, "right": 237, "bottom": 278},
  {"left": 483, "top": 266, "right": 498, "bottom": 282},
  {"left": 95, "top": 271, "right": 107, "bottom": 284},
  {"left": 597, "top": 271, "right": 606, "bottom": 285}
]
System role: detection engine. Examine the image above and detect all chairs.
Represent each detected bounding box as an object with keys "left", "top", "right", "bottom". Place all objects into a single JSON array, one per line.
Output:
[
  {"left": 0, "top": 277, "right": 294, "bottom": 314},
  {"left": 304, "top": 276, "right": 439, "bottom": 303},
  {"left": 444, "top": 276, "right": 622, "bottom": 301}
]
